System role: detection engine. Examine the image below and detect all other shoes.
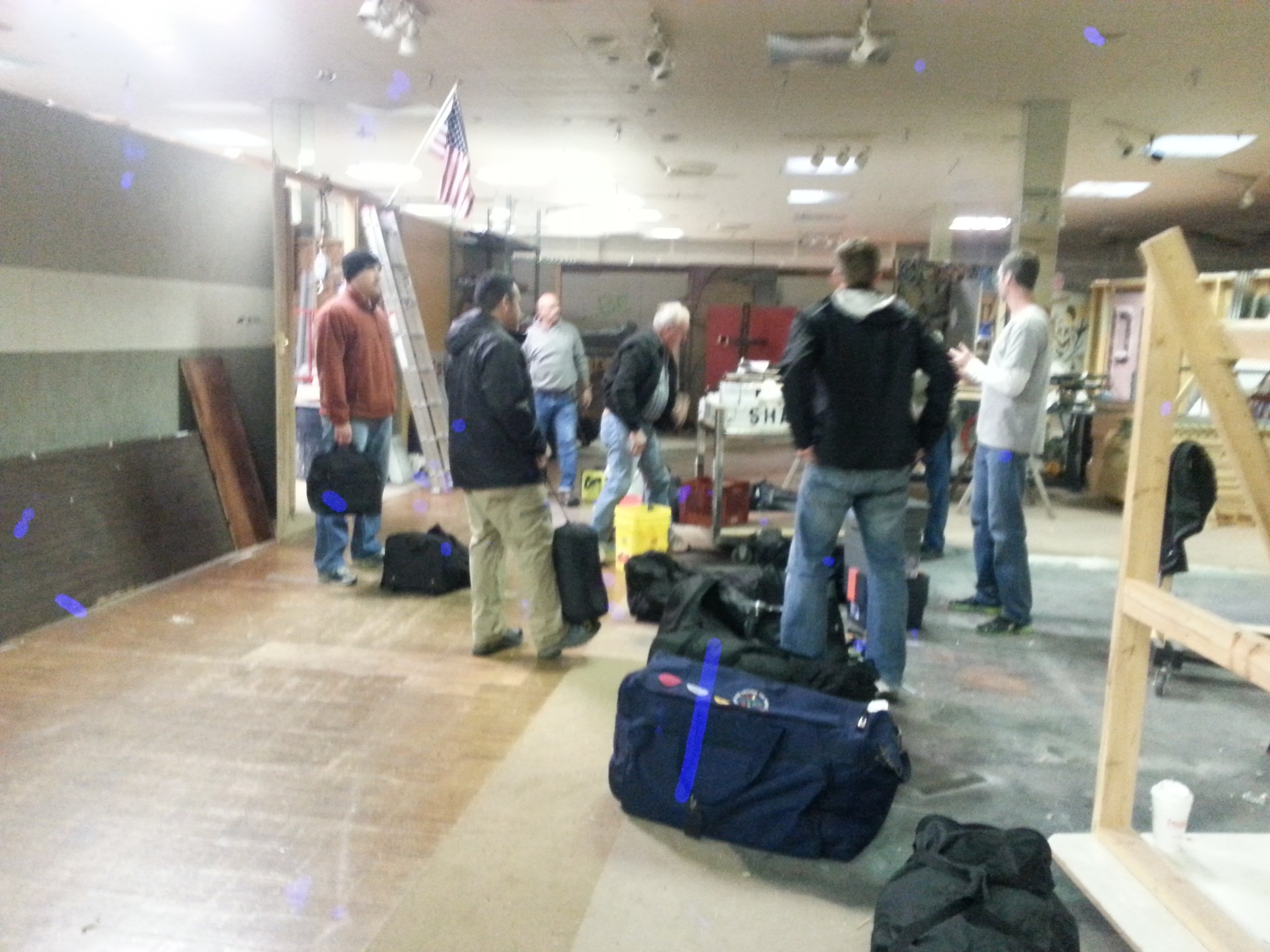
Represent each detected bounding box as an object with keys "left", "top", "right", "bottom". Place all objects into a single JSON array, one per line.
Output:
[
  {"left": 474, "top": 628, "right": 523, "bottom": 657},
  {"left": 919, "top": 545, "right": 943, "bottom": 560},
  {"left": 373, "top": 548, "right": 384, "bottom": 560},
  {"left": 543, "top": 622, "right": 600, "bottom": 659},
  {"left": 331, "top": 569, "right": 359, "bottom": 587},
  {"left": 977, "top": 616, "right": 1030, "bottom": 636},
  {"left": 948, "top": 596, "right": 1002, "bottom": 614}
]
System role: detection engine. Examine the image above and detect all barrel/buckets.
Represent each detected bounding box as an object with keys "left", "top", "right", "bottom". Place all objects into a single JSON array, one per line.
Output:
[{"left": 613, "top": 503, "right": 672, "bottom": 571}]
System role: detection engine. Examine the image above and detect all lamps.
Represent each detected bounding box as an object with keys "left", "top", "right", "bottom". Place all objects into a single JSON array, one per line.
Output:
[
  {"left": 854, "top": 138, "right": 871, "bottom": 169},
  {"left": 644, "top": 24, "right": 673, "bottom": 82},
  {"left": 357, "top": 0, "right": 426, "bottom": 58},
  {"left": 835, "top": 139, "right": 851, "bottom": 168},
  {"left": 811, "top": 141, "right": 826, "bottom": 168}
]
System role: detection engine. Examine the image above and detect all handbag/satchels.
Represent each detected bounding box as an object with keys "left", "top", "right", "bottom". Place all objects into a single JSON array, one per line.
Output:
[
  {"left": 552, "top": 519, "right": 608, "bottom": 622},
  {"left": 870, "top": 813, "right": 1081, "bottom": 951},
  {"left": 379, "top": 525, "right": 470, "bottom": 594},
  {"left": 607, "top": 654, "right": 910, "bottom": 860},
  {"left": 306, "top": 442, "right": 383, "bottom": 512},
  {"left": 623, "top": 534, "right": 878, "bottom": 703}
]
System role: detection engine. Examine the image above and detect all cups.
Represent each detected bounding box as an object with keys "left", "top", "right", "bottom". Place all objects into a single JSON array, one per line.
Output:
[{"left": 1150, "top": 785, "right": 1194, "bottom": 852}]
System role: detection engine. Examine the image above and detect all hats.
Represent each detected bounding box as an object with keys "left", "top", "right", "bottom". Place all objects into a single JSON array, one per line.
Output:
[{"left": 341, "top": 250, "right": 378, "bottom": 279}]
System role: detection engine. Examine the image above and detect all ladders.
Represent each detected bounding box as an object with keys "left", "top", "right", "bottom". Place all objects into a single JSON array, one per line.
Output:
[{"left": 361, "top": 204, "right": 453, "bottom": 495}]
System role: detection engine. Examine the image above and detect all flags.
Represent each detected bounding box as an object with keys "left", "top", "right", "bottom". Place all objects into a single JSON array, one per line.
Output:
[{"left": 427, "top": 88, "right": 475, "bottom": 219}]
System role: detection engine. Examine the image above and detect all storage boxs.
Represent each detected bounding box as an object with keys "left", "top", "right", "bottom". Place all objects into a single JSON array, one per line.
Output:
[{"left": 678, "top": 476, "right": 750, "bottom": 530}]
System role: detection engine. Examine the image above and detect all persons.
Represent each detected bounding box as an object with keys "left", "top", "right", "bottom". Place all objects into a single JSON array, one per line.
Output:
[
  {"left": 443, "top": 271, "right": 602, "bottom": 664},
  {"left": 310, "top": 246, "right": 397, "bottom": 585},
  {"left": 948, "top": 250, "right": 1051, "bottom": 632},
  {"left": 522, "top": 292, "right": 594, "bottom": 506},
  {"left": 899, "top": 327, "right": 954, "bottom": 560},
  {"left": 591, "top": 300, "right": 690, "bottom": 543},
  {"left": 776, "top": 236, "right": 960, "bottom": 702}
]
